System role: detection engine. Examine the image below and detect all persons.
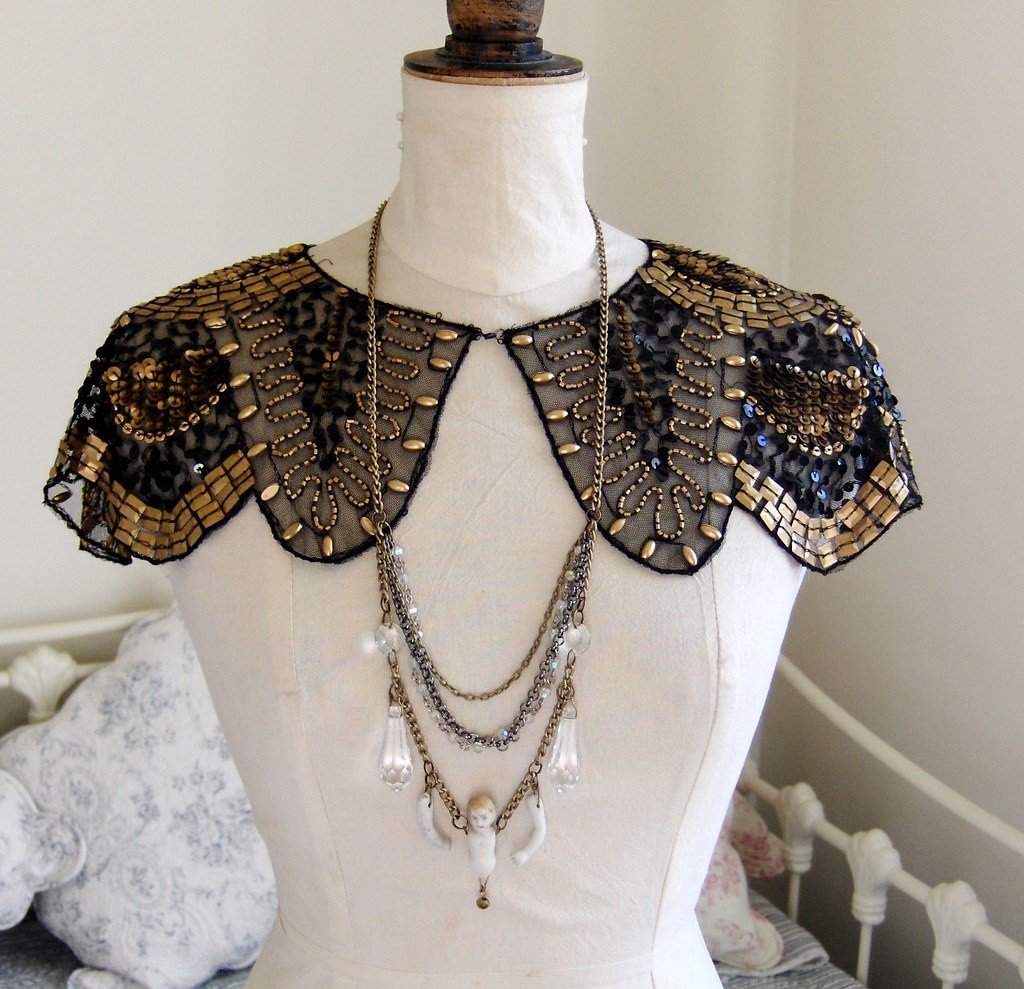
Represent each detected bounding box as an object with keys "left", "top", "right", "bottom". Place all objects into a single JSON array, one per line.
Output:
[{"left": 43, "top": 47, "right": 925, "bottom": 989}]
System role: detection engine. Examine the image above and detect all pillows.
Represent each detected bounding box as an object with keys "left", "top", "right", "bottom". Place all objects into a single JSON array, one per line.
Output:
[{"left": 0, "top": 600, "right": 277, "bottom": 989}]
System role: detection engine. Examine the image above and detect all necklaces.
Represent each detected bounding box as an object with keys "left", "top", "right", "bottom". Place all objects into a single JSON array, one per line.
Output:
[{"left": 363, "top": 198, "right": 610, "bottom": 906}]
